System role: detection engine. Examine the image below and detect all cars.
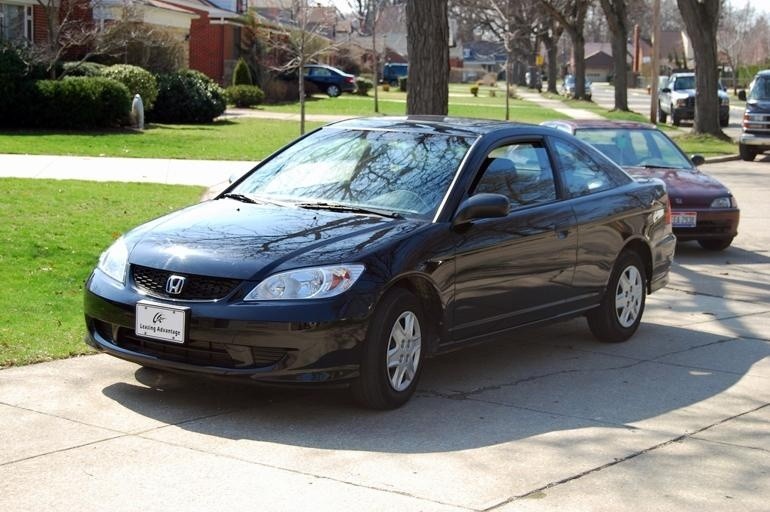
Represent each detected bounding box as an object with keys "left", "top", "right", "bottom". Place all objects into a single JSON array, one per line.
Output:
[
  {"left": 525, "top": 72, "right": 541, "bottom": 87},
  {"left": 384, "top": 63, "right": 408, "bottom": 86},
  {"left": 506, "top": 119, "right": 740, "bottom": 251},
  {"left": 562, "top": 74, "right": 592, "bottom": 99},
  {"left": 739, "top": 69, "right": 770, "bottom": 160},
  {"left": 304, "top": 64, "right": 358, "bottom": 97},
  {"left": 84, "top": 114, "right": 677, "bottom": 412}
]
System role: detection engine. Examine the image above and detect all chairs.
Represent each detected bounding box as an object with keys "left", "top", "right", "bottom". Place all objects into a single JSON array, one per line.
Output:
[
  {"left": 461, "top": 153, "right": 590, "bottom": 213},
  {"left": 589, "top": 144, "right": 633, "bottom": 166}
]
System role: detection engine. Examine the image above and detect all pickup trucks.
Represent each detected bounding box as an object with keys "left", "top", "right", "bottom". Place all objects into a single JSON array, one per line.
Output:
[{"left": 656, "top": 72, "right": 729, "bottom": 126}]
